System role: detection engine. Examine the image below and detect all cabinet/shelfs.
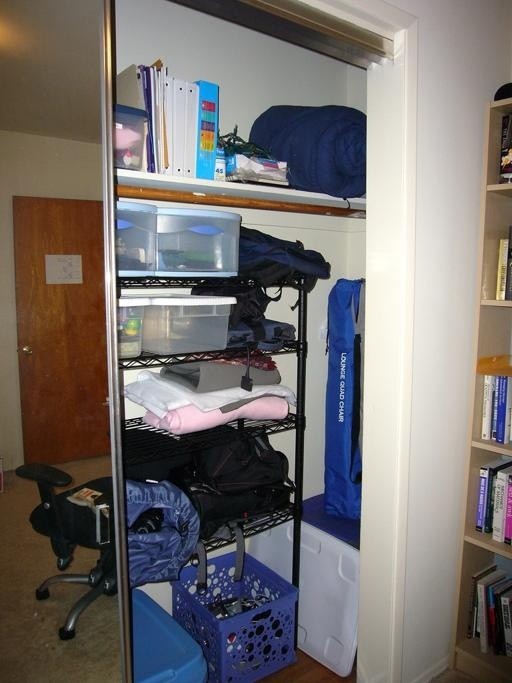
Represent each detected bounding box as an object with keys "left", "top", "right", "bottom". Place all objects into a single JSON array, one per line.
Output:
[
  {"left": 455, "top": 98, "right": 512, "bottom": 680},
  {"left": 99, "top": 3, "right": 380, "bottom": 682}
]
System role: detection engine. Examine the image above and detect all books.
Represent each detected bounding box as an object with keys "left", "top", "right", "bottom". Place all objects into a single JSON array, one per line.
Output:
[
  {"left": 500, "top": 108, "right": 511, "bottom": 182},
  {"left": 113, "top": 57, "right": 289, "bottom": 188},
  {"left": 474, "top": 455, "right": 511, "bottom": 546},
  {"left": 495, "top": 225, "right": 510, "bottom": 302},
  {"left": 477, "top": 370, "right": 511, "bottom": 446},
  {"left": 471, "top": 563, "right": 512, "bottom": 662}
]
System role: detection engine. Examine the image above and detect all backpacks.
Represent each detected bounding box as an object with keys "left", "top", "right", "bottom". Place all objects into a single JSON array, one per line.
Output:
[
  {"left": 190, "top": 286, "right": 297, "bottom": 351},
  {"left": 237, "top": 226, "right": 332, "bottom": 295}
]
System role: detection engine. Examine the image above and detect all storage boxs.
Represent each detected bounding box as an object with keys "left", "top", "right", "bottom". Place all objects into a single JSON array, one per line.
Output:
[
  {"left": 118, "top": 292, "right": 237, "bottom": 359},
  {"left": 251, "top": 493, "right": 359, "bottom": 678},
  {"left": 117, "top": 198, "right": 241, "bottom": 280},
  {"left": 127, "top": 590, "right": 207, "bottom": 683},
  {"left": 170, "top": 552, "right": 299, "bottom": 679}
]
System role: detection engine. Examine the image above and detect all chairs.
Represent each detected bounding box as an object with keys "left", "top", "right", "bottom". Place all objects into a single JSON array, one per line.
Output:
[{"left": 14, "top": 463, "right": 118, "bottom": 643}]
